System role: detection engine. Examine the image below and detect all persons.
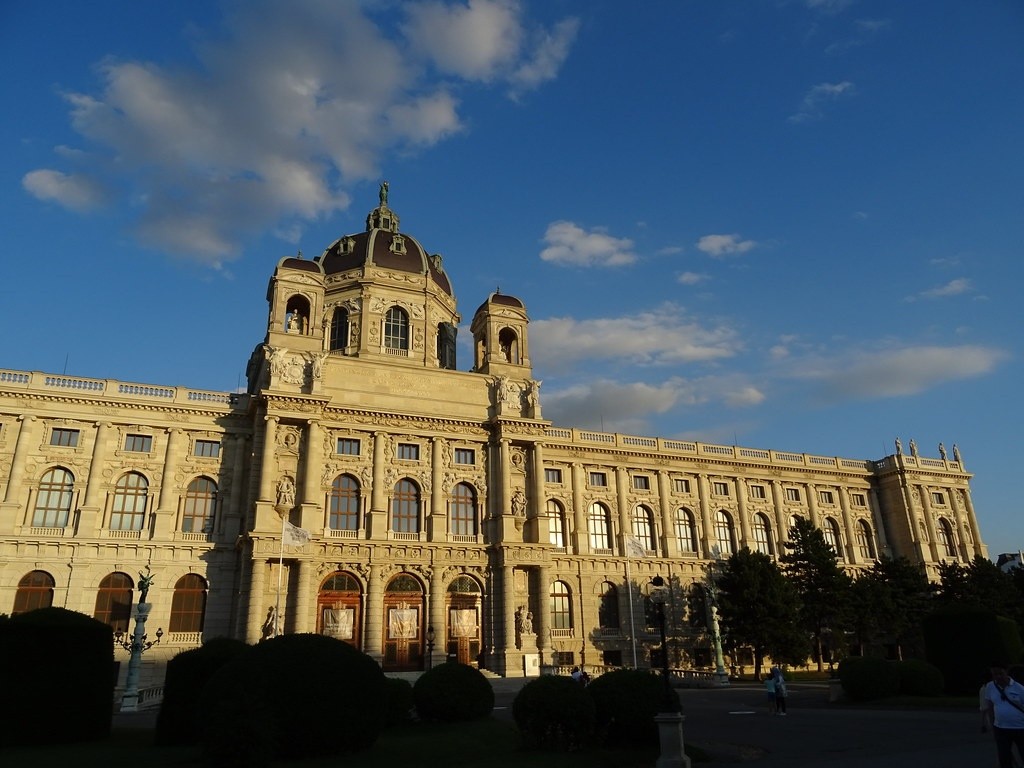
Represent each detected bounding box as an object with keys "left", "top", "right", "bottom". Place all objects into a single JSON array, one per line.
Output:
[
  {"left": 286, "top": 308, "right": 301, "bottom": 329},
  {"left": 498, "top": 341, "right": 511, "bottom": 361},
  {"left": 979, "top": 678, "right": 994, "bottom": 735},
  {"left": 493, "top": 375, "right": 541, "bottom": 410},
  {"left": 278, "top": 477, "right": 298, "bottom": 507},
  {"left": 572, "top": 666, "right": 592, "bottom": 688},
  {"left": 283, "top": 434, "right": 297, "bottom": 452},
  {"left": 264, "top": 604, "right": 284, "bottom": 638},
  {"left": 511, "top": 491, "right": 528, "bottom": 516},
  {"left": 517, "top": 605, "right": 534, "bottom": 632},
  {"left": 267, "top": 347, "right": 328, "bottom": 388},
  {"left": 984, "top": 662, "right": 1024, "bottom": 768},
  {"left": 513, "top": 455, "right": 525, "bottom": 470},
  {"left": 764, "top": 666, "right": 789, "bottom": 717}
]
